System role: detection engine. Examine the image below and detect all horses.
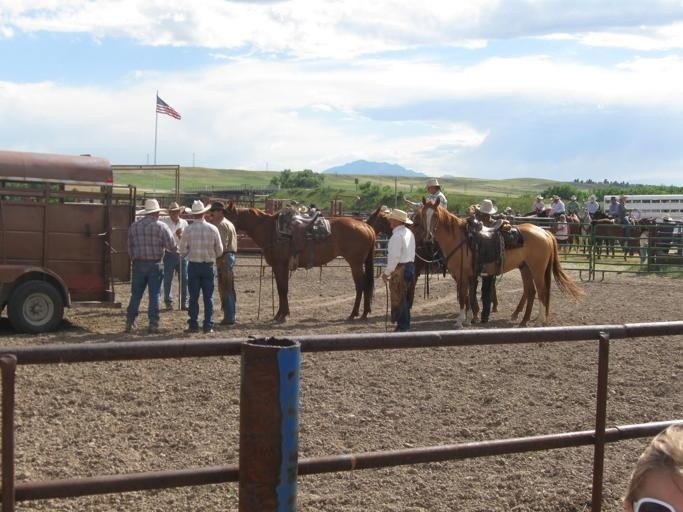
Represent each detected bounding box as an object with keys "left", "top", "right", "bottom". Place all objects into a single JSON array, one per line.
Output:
[
  {"left": 587, "top": 218, "right": 656, "bottom": 260},
  {"left": 566, "top": 209, "right": 582, "bottom": 254},
  {"left": 515, "top": 207, "right": 571, "bottom": 257},
  {"left": 366, "top": 205, "right": 498, "bottom": 324},
  {"left": 223, "top": 202, "right": 375, "bottom": 321},
  {"left": 414, "top": 196, "right": 585, "bottom": 326}
]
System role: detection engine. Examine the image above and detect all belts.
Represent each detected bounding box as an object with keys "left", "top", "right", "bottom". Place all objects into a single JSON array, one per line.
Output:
[{"left": 133, "top": 258, "right": 161, "bottom": 264}]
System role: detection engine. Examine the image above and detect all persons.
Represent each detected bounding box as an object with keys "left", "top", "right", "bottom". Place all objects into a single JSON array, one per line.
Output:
[
  {"left": 550, "top": 195, "right": 565, "bottom": 215},
  {"left": 640, "top": 228, "right": 648, "bottom": 269},
  {"left": 159, "top": 202, "right": 190, "bottom": 311},
  {"left": 309, "top": 205, "right": 318, "bottom": 217},
  {"left": 586, "top": 196, "right": 598, "bottom": 215},
  {"left": 209, "top": 201, "right": 236, "bottom": 326},
  {"left": 124, "top": 199, "right": 177, "bottom": 335},
  {"left": 553, "top": 215, "right": 570, "bottom": 259},
  {"left": 400, "top": 178, "right": 448, "bottom": 210},
  {"left": 382, "top": 208, "right": 416, "bottom": 331},
  {"left": 607, "top": 198, "right": 618, "bottom": 221},
  {"left": 566, "top": 195, "right": 580, "bottom": 214},
  {"left": 179, "top": 200, "right": 223, "bottom": 334},
  {"left": 534, "top": 194, "right": 544, "bottom": 214},
  {"left": 656, "top": 213, "right": 676, "bottom": 254},
  {"left": 615, "top": 194, "right": 634, "bottom": 240},
  {"left": 471, "top": 200, "right": 495, "bottom": 326}
]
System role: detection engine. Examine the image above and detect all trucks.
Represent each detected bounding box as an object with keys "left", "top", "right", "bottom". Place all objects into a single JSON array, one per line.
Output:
[{"left": 0, "top": 149, "right": 136, "bottom": 333}]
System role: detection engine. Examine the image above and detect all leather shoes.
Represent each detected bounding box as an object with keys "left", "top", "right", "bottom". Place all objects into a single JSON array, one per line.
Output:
[{"left": 217, "top": 320, "right": 233, "bottom": 326}]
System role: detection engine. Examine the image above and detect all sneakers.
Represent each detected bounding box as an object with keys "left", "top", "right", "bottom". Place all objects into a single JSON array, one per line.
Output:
[
  {"left": 161, "top": 306, "right": 174, "bottom": 313},
  {"left": 148, "top": 328, "right": 161, "bottom": 336},
  {"left": 203, "top": 329, "right": 214, "bottom": 334},
  {"left": 184, "top": 328, "right": 199, "bottom": 335},
  {"left": 123, "top": 330, "right": 137, "bottom": 338},
  {"left": 179, "top": 305, "right": 189, "bottom": 311}
]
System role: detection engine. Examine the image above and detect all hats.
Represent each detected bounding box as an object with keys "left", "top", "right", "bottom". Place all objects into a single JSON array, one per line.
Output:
[
  {"left": 552, "top": 194, "right": 561, "bottom": 201},
  {"left": 165, "top": 202, "right": 185, "bottom": 213},
  {"left": 137, "top": 198, "right": 167, "bottom": 215},
  {"left": 570, "top": 195, "right": 577, "bottom": 201},
  {"left": 426, "top": 179, "right": 440, "bottom": 187},
  {"left": 662, "top": 213, "right": 673, "bottom": 222},
  {"left": 382, "top": 209, "right": 413, "bottom": 225},
  {"left": 209, "top": 202, "right": 226, "bottom": 210},
  {"left": 537, "top": 194, "right": 544, "bottom": 200},
  {"left": 184, "top": 200, "right": 212, "bottom": 215},
  {"left": 588, "top": 194, "right": 598, "bottom": 202},
  {"left": 474, "top": 199, "right": 498, "bottom": 215},
  {"left": 614, "top": 194, "right": 628, "bottom": 201}
]
